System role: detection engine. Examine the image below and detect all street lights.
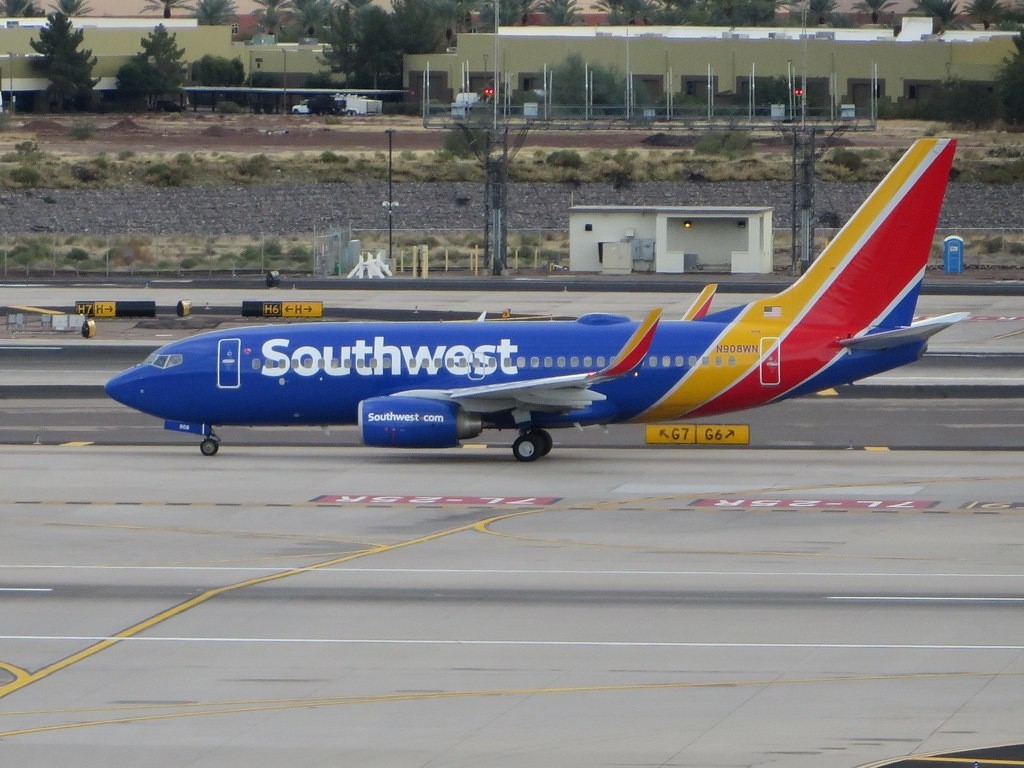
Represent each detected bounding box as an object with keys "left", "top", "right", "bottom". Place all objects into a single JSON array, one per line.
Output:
[{"left": 384, "top": 127, "right": 394, "bottom": 257}]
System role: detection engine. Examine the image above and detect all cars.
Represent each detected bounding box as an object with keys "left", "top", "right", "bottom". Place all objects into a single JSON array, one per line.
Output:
[{"left": 291, "top": 99, "right": 310, "bottom": 114}]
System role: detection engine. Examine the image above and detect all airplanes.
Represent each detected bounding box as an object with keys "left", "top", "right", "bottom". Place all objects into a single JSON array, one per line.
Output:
[{"left": 104, "top": 136, "right": 969, "bottom": 462}]
